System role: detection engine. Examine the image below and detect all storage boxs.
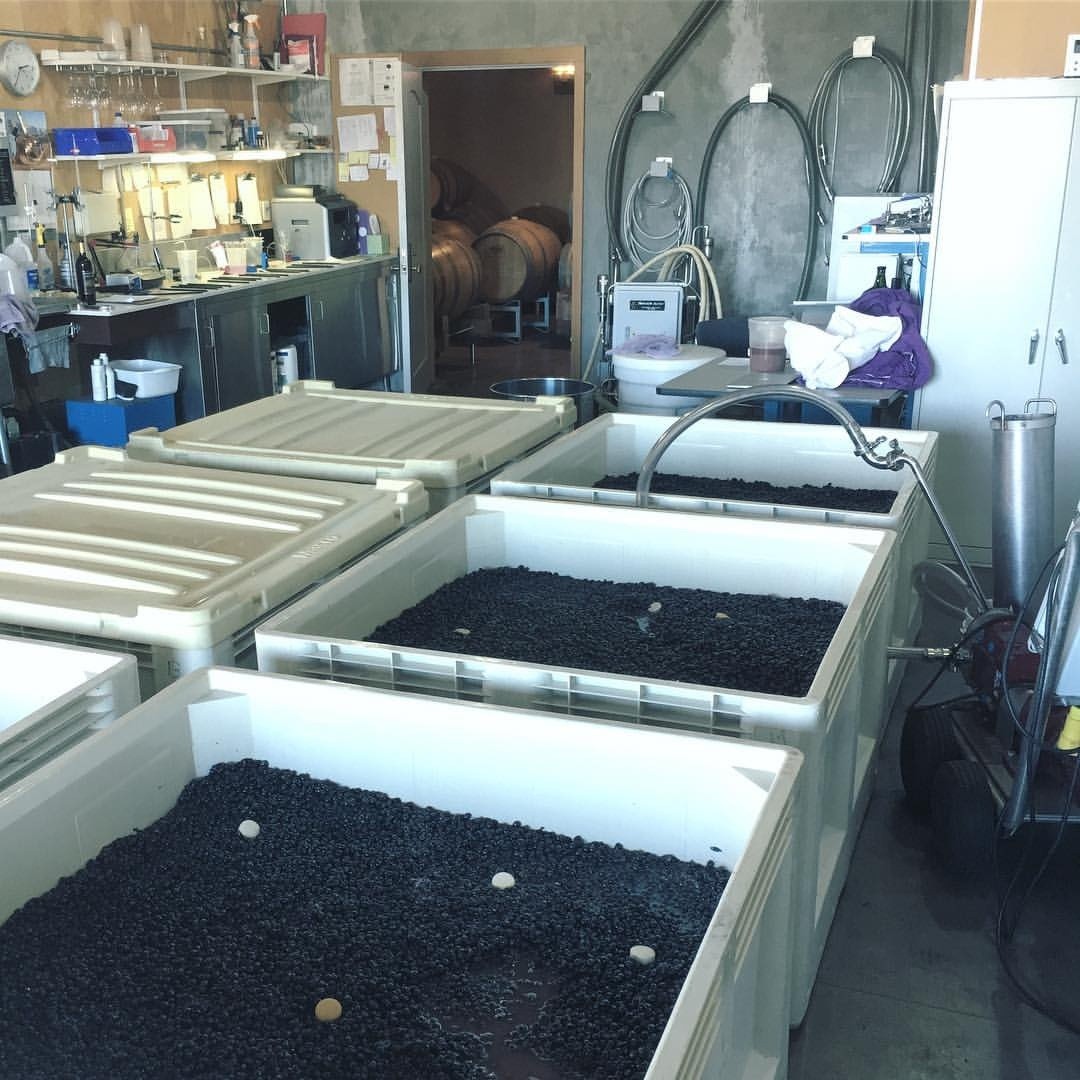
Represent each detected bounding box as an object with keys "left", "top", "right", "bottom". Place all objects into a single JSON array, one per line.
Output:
[
  {"left": 112, "top": 358, "right": 182, "bottom": 397},
  {"left": 55, "top": 130, "right": 136, "bottom": 158},
  {"left": 0, "top": 380, "right": 939, "bottom": 1080},
  {"left": 130, "top": 127, "right": 181, "bottom": 152}
]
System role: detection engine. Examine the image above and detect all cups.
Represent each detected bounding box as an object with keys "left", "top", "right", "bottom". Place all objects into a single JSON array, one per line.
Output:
[
  {"left": 225, "top": 248, "right": 248, "bottom": 274},
  {"left": 175, "top": 250, "right": 199, "bottom": 281},
  {"left": 747, "top": 316, "right": 789, "bottom": 372},
  {"left": 130, "top": 22, "right": 153, "bottom": 62},
  {"left": 103, "top": 19, "right": 126, "bottom": 59},
  {"left": 240, "top": 237, "right": 264, "bottom": 272}
]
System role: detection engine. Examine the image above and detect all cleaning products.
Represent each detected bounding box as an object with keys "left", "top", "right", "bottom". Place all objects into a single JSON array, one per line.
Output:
[{"left": 89, "top": 352, "right": 116, "bottom": 402}]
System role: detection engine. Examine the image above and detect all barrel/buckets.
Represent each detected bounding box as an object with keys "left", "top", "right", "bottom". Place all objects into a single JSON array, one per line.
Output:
[
  {"left": 614, "top": 343, "right": 726, "bottom": 418},
  {"left": 426, "top": 149, "right": 575, "bottom": 322},
  {"left": 489, "top": 377, "right": 598, "bottom": 431}
]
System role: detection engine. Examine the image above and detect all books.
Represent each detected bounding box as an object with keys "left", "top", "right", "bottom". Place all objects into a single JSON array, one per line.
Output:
[{"left": 282, "top": 12, "right": 328, "bottom": 75}]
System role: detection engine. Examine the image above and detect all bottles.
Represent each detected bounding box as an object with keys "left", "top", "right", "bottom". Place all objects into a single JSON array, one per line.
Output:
[
  {"left": 91, "top": 353, "right": 115, "bottom": 400},
  {"left": 75, "top": 240, "right": 95, "bottom": 305},
  {"left": 0, "top": 253, "right": 22, "bottom": 296},
  {"left": 273, "top": 48, "right": 281, "bottom": 71},
  {"left": 111, "top": 112, "right": 139, "bottom": 153},
  {"left": 277, "top": 344, "right": 299, "bottom": 392},
  {"left": 231, "top": 113, "right": 265, "bottom": 149},
  {"left": 4, "top": 238, "right": 39, "bottom": 293},
  {"left": 151, "top": 122, "right": 168, "bottom": 141},
  {"left": 35, "top": 244, "right": 53, "bottom": 289}
]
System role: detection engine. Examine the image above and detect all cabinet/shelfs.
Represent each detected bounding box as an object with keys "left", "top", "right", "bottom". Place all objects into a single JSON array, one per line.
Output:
[
  {"left": 911, "top": 77, "right": 1080, "bottom": 571},
  {"left": 41, "top": 53, "right": 324, "bottom": 167}
]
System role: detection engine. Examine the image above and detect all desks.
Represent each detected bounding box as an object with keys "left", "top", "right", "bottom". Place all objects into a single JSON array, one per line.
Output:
[{"left": 657, "top": 352, "right": 902, "bottom": 423}]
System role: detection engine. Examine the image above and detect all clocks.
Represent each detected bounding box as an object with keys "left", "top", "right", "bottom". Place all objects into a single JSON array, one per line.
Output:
[{"left": 1, "top": 39, "right": 41, "bottom": 96}]
